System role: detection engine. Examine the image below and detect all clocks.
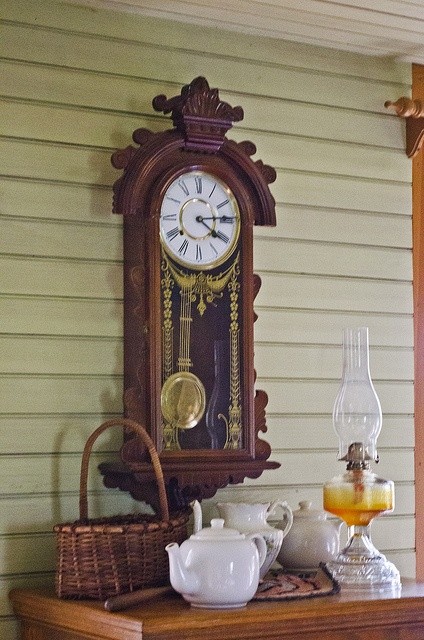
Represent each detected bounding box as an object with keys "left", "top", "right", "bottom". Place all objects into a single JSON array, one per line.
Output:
[{"left": 99, "top": 76, "right": 278, "bottom": 516}]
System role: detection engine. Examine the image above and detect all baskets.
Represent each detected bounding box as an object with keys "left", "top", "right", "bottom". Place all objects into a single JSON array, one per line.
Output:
[{"left": 52, "top": 416, "right": 190, "bottom": 599}]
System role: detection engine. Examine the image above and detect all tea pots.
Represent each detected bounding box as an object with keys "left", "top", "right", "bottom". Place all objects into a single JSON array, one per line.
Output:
[
  {"left": 275, "top": 503, "right": 353, "bottom": 574},
  {"left": 166, "top": 519, "right": 267, "bottom": 610},
  {"left": 190, "top": 500, "right": 292, "bottom": 583}
]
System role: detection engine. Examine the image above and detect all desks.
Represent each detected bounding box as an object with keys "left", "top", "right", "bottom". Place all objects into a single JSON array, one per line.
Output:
[{"left": 8, "top": 587, "right": 424, "bottom": 640}]
